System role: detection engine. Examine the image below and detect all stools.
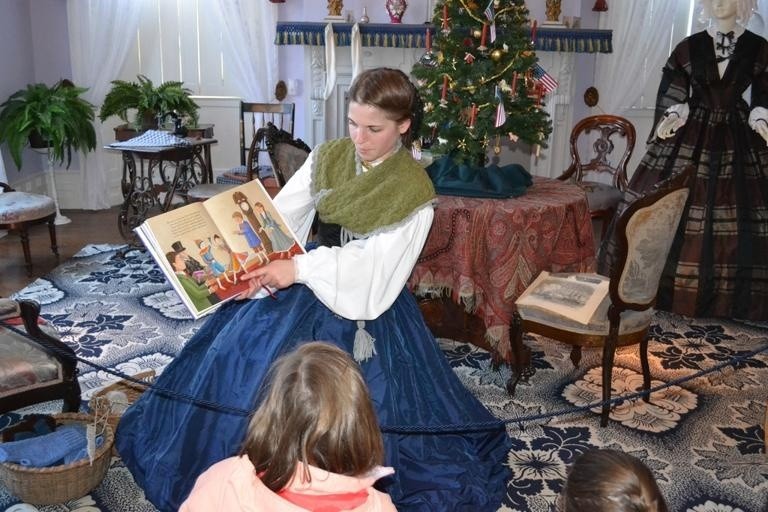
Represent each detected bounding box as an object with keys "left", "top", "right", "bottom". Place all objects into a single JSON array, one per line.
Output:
[
  {"left": 185, "top": 183, "right": 242, "bottom": 202},
  {"left": 2, "top": 182, "right": 60, "bottom": 276},
  {"left": 1, "top": 297, "right": 81, "bottom": 427}
]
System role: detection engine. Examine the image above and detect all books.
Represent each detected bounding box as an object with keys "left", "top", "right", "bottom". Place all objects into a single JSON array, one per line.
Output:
[{"left": 133, "top": 177, "right": 310, "bottom": 320}]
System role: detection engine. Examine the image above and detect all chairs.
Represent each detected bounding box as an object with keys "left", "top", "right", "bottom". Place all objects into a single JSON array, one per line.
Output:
[
  {"left": 556, "top": 114, "right": 637, "bottom": 194},
  {"left": 216, "top": 99, "right": 295, "bottom": 185},
  {"left": 265, "top": 121, "right": 315, "bottom": 232},
  {"left": 505, "top": 165, "right": 693, "bottom": 428}
]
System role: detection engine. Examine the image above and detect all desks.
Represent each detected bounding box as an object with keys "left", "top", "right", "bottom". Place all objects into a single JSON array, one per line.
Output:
[
  {"left": 103, "top": 137, "right": 216, "bottom": 241},
  {"left": 404, "top": 172, "right": 599, "bottom": 365}
]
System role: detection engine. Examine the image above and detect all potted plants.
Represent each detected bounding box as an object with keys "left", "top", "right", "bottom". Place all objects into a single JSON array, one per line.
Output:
[
  {"left": 1, "top": 77, "right": 98, "bottom": 171},
  {"left": 98, "top": 69, "right": 200, "bottom": 141}
]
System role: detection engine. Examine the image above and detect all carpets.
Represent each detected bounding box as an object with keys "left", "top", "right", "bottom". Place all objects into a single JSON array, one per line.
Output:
[{"left": 1, "top": 239, "right": 768, "bottom": 510}]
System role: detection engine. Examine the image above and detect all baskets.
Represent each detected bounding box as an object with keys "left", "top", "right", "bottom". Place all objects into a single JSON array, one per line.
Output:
[{"left": 0, "top": 412, "right": 114, "bottom": 506}]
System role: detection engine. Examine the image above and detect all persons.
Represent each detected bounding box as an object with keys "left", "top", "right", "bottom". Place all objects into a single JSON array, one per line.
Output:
[
  {"left": 109, "top": 67, "right": 512, "bottom": 510},
  {"left": 595, "top": 0, "right": 768, "bottom": 325},
  {"left": 171, "top": 338, "right": 401, "bottom": 511},
  {"left": 553, "top": 448, "right": 668, "bottom": 512}
]
